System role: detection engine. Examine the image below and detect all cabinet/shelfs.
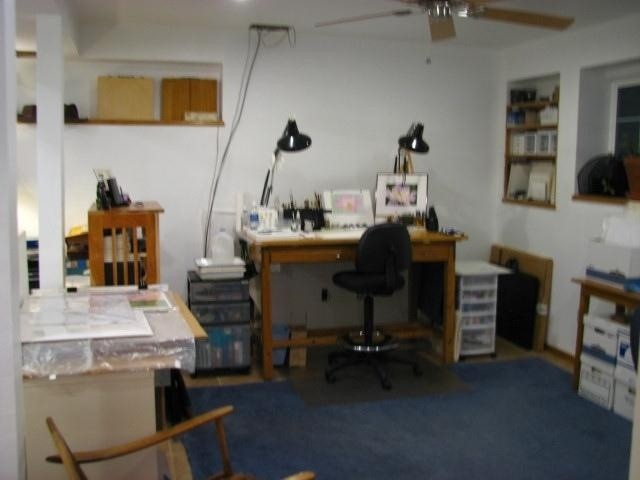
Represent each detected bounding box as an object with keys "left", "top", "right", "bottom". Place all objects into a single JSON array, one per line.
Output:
[
  {"left": 88, "top": 199, "right": 164, "bottom": 288},
  {"left": 502, "top": 100, "right": 559, "bottom": 210}
]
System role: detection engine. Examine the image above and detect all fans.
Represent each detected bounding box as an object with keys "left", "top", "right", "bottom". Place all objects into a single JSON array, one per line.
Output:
[{"left": 313, "top": 0, "right": 576, "bottom": 41}]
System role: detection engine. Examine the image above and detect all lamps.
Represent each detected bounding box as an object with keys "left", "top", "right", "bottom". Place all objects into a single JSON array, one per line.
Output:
[
  {"left": 259, "top": 114, "right": 312, "bottom": 206},
  {"left": 394, "top": 119, "right": 430, "bottom": 171}
]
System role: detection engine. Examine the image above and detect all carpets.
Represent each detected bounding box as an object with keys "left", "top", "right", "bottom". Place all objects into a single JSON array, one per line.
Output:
[{"left": 174, "top": 357, "right": 632, "bottom": 480}]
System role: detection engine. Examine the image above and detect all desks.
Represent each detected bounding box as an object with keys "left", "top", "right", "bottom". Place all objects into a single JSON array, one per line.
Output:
[
  {"left": 570, "top": 276, "right": 639, "bottom": 385},
  {"left": 240, "top": 226, "right": 470, "bottom": 379},
  {"left": 19, "top": 290, "right": 209, "bottom": 479}
]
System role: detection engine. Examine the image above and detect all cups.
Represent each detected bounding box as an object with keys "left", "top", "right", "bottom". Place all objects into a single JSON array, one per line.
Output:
[{"left": 304, "top": 220, "right": 315, "bottom": 231}]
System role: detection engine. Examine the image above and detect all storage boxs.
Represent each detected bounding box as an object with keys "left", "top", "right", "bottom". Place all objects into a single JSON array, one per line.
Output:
[
  {"left": 580, "top": 314, "right": 618, "bottom": 366},
  {"left": 160, "top": 77, "right": 218, "bottom": 123},
  {"left": 454, "top": 273, "right": 500, "bottom": 361},
  {"left": 577, "top": 354, "right": 614, "bottom": 411},
  {"left": 96, "top": 77, "right": 154, "bottom": 123},
  {"left": 187, "top": 273, "right": 254, "bottom": 378},
  {"left": 612, "top": 366, "right": 635, "bottom": 422},
  {"left": 491, "top": 244, "right": 553, "bottom": 352},
  {"left": 614, "top": 326, "right": 635, "bottom": 372},
  {"left": 587, "top": 237, "right": 640, "bottom": 282}
]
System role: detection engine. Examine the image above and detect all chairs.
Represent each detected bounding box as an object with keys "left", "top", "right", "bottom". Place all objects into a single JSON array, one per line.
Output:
[
  {"left": 45, "top": 406, "right": 317, "bottom": 480},
  {"left": 325, "top": 223, "right": 424, "bottom": 392}
]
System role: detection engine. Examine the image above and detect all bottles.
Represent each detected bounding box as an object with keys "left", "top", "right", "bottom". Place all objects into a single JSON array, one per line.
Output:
[
  {"left": 211, "top": 228, "right": 234, "bottom": 263},
  {"left": 249, "top": 200, "right": 259, "bottom": 232}
]
